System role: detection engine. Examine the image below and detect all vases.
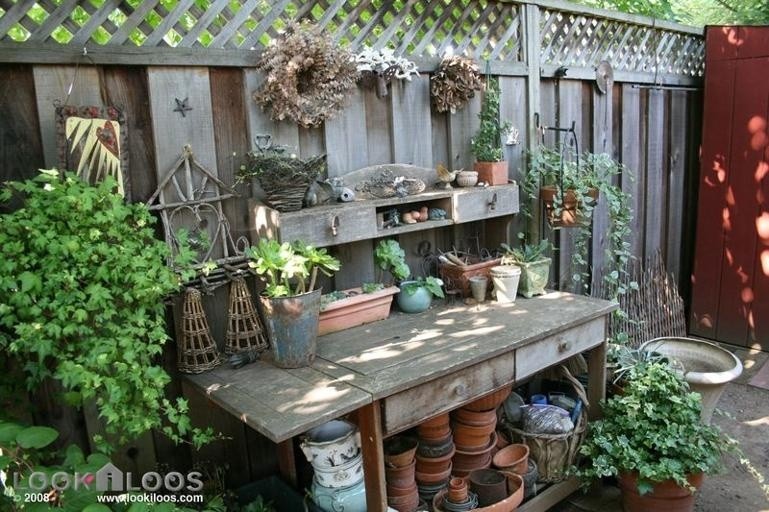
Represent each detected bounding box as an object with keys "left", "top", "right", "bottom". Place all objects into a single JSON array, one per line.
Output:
[
  {"left": 639, "top": 337, "right": 743, "bottom": 428},
  {"left": 298, "top": 381, "right": 540, "bottom": 512}
]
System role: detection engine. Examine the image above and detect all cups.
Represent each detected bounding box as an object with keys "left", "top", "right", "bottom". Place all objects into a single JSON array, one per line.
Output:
[
  {"left": 469, "top": 275, "right": 489, "bottom": 303},
  {"left": 489, "top": 264, "right": 522, "bottom": 304}
]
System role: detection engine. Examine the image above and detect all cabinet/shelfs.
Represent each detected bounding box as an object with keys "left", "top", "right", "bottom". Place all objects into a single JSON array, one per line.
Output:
[{"left": 172, "top": 163, "right": 620, "bottom": 511}]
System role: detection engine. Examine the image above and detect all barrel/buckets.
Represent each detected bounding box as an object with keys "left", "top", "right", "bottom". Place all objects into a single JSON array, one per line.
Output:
[{"left": 259, "top": 284, "right": 322, "bottom": 371}]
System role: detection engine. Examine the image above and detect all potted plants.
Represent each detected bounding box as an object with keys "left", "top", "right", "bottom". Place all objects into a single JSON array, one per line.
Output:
[
  {"left": 244, "top": 233, "right": 445, "bottom": 369},
  {"left": 579, "top": 361, "right": 734, "bottom": 511},
  {"left": 501, "top": 142, "right": 635, "bottom": 299}
]
